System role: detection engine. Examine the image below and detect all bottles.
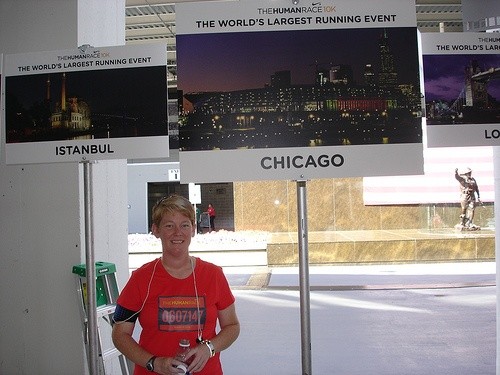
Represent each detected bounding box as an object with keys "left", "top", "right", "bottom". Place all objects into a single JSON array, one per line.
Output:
[{"left": 174, "top": 339, "right": 193, "bottom": 375}]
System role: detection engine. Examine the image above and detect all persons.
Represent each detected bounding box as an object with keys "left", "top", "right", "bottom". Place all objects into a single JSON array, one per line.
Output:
[
  {"left": 455, "top": 167, "right": 481, "bottom": 232},
  {"left": 193, "top": 203, "right": 216, "bottom": 234},
  {"left": 112, "top": 194, "right": 240, "bottom": 375}
]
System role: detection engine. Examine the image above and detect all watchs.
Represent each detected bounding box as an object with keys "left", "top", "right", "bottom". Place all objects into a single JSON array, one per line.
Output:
[{"left": 146, "top": 356, "right": 157, "bottom": 372}]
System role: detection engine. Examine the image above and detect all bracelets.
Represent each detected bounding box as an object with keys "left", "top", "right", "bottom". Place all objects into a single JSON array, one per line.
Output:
[{"left": 200, "top": 339, "right": 215, "bottom": 358}]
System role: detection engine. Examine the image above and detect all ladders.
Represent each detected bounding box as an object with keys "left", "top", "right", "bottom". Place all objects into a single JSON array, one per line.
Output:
[{"left": 72, "top": 260, "right": 137, "bottom": 375}]
